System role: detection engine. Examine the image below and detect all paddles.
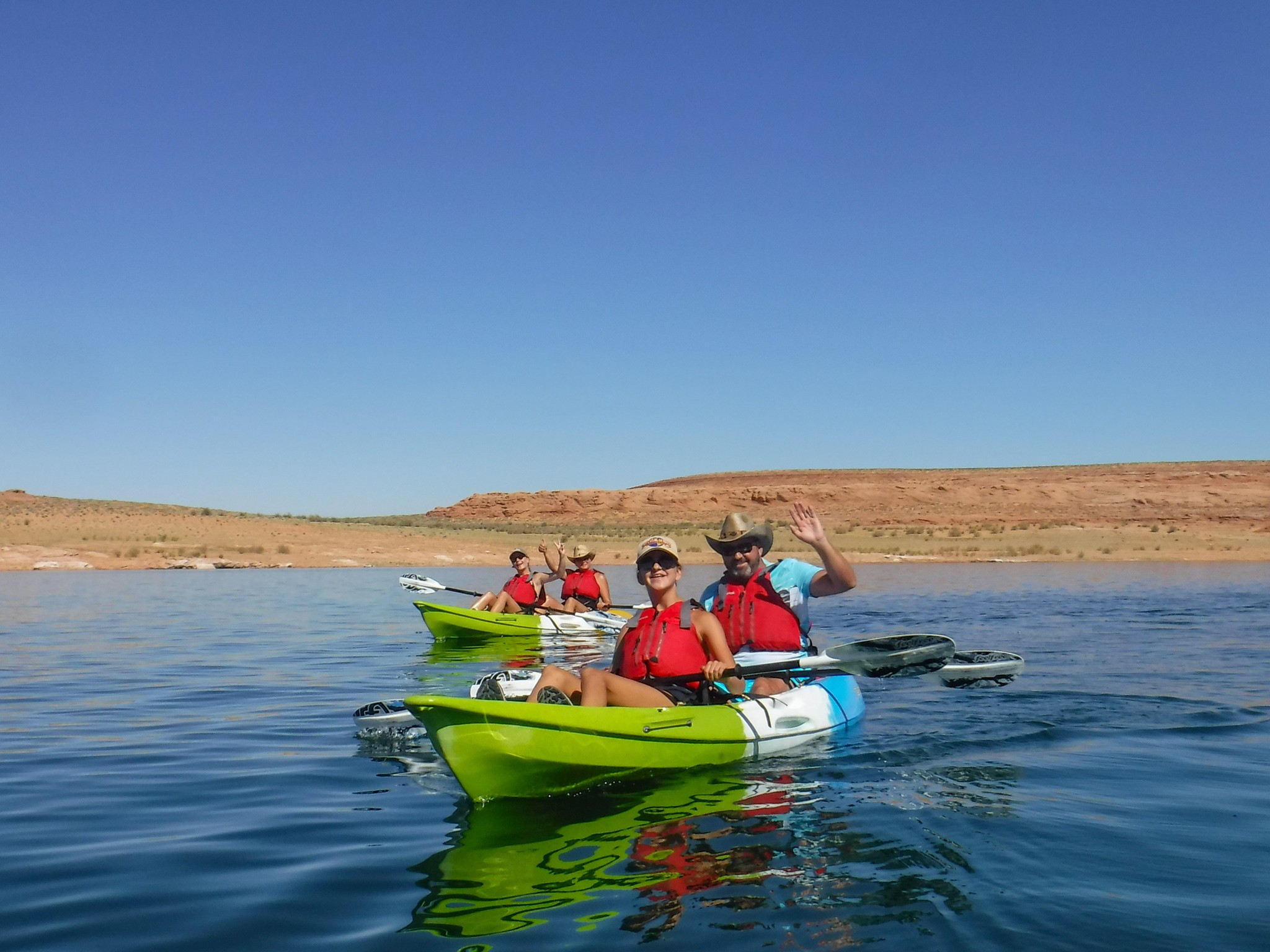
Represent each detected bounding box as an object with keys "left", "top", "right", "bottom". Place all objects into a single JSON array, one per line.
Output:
[
  {"left": 470, "top": 649, "right": 1024, "bottom": 703},
  {"left": 584, "top": 600, "right": 653, "bottom": 610},
  {"left": 352, "top": 631, "right": 957, "bottom": 734},
  {"left": 399, "top": 572, "right": 629, "bottom": 629}
]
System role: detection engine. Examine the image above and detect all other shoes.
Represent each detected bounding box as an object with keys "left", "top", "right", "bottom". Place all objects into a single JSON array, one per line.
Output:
[
  {"left": 476, "top": 679, "right": 506, "bottom": 701},
  {"left": 537, "top": 686, "right": 574, "bottom": 706}
]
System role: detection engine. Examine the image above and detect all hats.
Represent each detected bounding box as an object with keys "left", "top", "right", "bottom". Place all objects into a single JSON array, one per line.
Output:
[
  {"left": 705, "top": 513, "right": 774, "bottom": 557},
  {"left": 510, "top": 549, "right": 529, "bottom": 565},
  {"left": 635, "top": 535, "right": 678, "bottom": 564},
  {"left": 566, "top": 545, "right": 596, "bottom": 563}
]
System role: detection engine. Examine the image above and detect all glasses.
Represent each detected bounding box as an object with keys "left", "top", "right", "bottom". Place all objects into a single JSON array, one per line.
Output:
[
  {"left": 720, "top": 541, "right": 760, "bottom": 557},
  {"left": 511, "top": 555, "right": 527, "bottom": 565},
  {"left": 638, "top": 555, "right": 679, "bottom": 570}
]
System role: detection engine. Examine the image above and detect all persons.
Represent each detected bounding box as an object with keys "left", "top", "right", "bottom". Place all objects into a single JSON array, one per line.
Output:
[
  {"left": 697, "top": 502, "right": 856, "bottom": 704},
  {"left": 468, "top": 538, "right": 566, "bottom": 615},
  {"left": 474, "top": 536, "right": 746, "bottom": 707},
  {"left": 538, "top": 538, "right": 612, "bottom": 616}
]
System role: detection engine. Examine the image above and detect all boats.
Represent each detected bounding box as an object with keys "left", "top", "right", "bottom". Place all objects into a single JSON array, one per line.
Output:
[
  {"left": 413, "top": 600, "right": 634, "bottom": 640},
  {"left": 402, "top": 669, "right": 868, "bottom": 804}
]
